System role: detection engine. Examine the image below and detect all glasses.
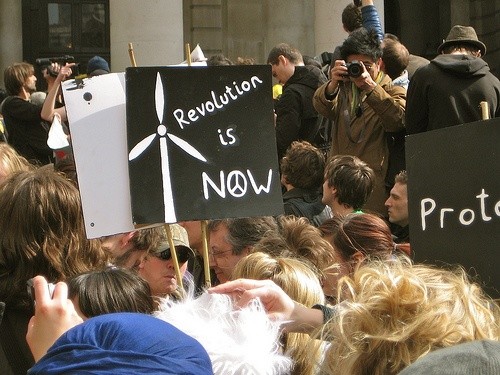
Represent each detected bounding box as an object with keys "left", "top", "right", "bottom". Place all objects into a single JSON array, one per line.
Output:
[
  {"left": 160, "top": 249, "right": 189, "bottom": 263},
  {"left": 210, "top": 248, "right": 233, "bottom": 261}
]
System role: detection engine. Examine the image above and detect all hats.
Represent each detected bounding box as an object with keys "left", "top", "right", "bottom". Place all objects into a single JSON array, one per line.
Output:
[
  {"left": 437, "top": 25, "right": 486, "bottom": 59},
  {"left": 86, "top": 56, "right": 110, "bottom": 73},
  {"left": 148, "top": 223, "right": 196, "bottom": 260}
]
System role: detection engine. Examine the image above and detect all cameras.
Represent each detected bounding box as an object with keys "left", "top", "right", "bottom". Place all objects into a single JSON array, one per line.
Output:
[
  {"left": 25, "top": 279, "right": 55, "bottom": 313},
  {"left": 36, "top": 55, "right": 74, "bottom": 76},
  {"left": 339, "top": 61, "right": 363, "bottom": 78}
]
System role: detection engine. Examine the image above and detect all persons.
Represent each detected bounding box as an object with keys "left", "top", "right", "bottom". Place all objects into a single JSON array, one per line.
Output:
[
  {"left": 0, "top": 55, "right": 110, "bottom": 185},
  {"left": 0, "top": 167, "right": 294, "bottom": 375},
  {"left": 322, "top": 154, "right": 376, "bottom": 218},
  {"left": 210, "top": 214, "right": 280, "bottom": 284},
  {"left": 405, "top": 25, "right": 500, "bottom": 135},
  {"left": 313, "top": 27, "right": 407, "bottom": 208},
  {"left": 101, "top": 219, "right": 213, "bottom": 311},
  {"left": 67, "top": 270, "right": 154, "bottom": 321},
  {"left": 233, "top": 214, "right": 500, "bottom": 375},
  {"left": 384, "top": 170, "right": 409, "bottom": 244},
  {"left": 281, "top": 141, "right": 333, "bottom": 228},
  {"left": 268, "top": 43, "right": 334, "bottom": 157},
  {"left": 331, "top": 0, "right": 385, "bottom": 67},
  {"left": 26, "top": 275, "right": 84, "bottom": 364}
]
